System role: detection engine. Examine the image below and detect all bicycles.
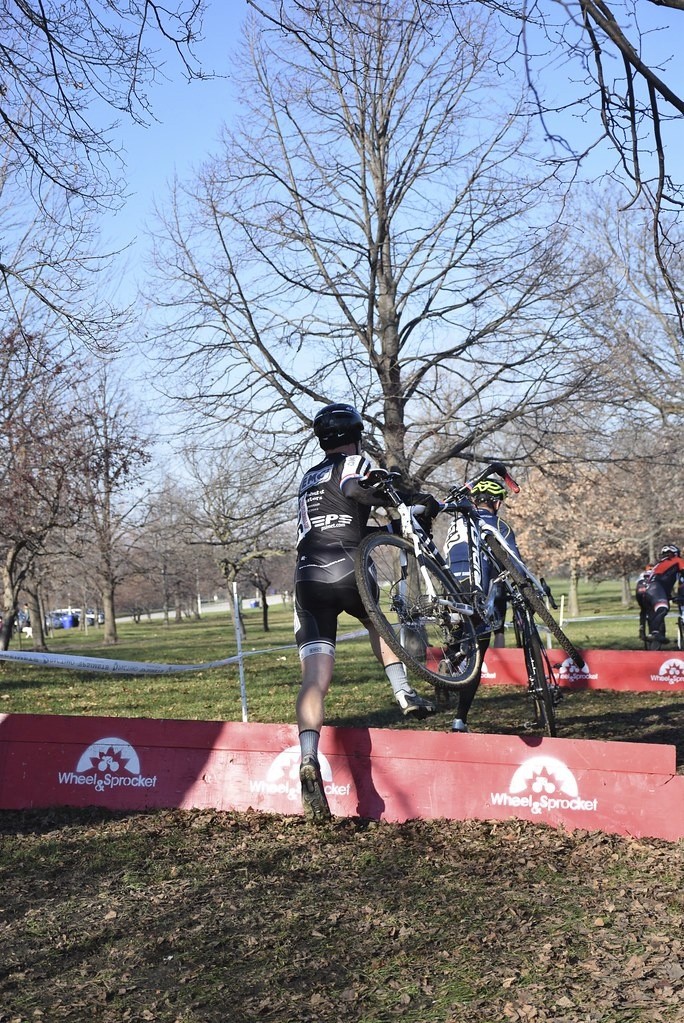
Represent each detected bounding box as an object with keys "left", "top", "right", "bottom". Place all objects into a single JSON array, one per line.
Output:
[
  {"left": 505, "top": 576, "right": 559, "bottom": 737},
  {"left": 354, "top": 468, "right": 586, "bottom": 692},
  {"left": 670, "top": 597, "right": 684, "bottom": 650},
  {"left": 641, "top": 610, "right": 660, "bottom": 650}
]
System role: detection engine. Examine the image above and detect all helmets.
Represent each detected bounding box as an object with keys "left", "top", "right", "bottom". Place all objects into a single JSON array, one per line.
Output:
[
  {"left": 643, "top": 572, "right": 651, "bottom": 576},
  {"left": 314, "top": 403, "right": 364, "bottom": 435},
  {"left": 660, "top": 545, "right": 680, "bottom": 554},
  {"left": 468, "top": 478, "right": 507, "bottom": 500}
]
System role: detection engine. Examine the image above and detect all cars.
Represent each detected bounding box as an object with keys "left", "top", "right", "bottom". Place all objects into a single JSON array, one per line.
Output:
[{"left": 0, "top": 608, "right": 104, "bottom": 634}]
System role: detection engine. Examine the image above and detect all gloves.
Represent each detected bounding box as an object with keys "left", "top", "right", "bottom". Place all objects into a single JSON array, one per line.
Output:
[{"left": 411, "top": 492, "right": 440, "bottom": 520}]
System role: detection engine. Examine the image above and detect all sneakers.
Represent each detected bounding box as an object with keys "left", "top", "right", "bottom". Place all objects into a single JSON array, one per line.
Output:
[
  {"left": 300, "top": 758, "right": 332, "bottom": 823},
  {"left": 452, "top": 718, "right": 468, "bottom": 732},
  {"left": 639, "top": 626, "right": 646, "bottom": 641},
  {"left": 435, "top": 659, "right": 451, "bottom": 703},
  {"left": 647, "top": 633, "right": 670, "bottom": 644},
  {"left": 394, "top": 689, "right": 436, "bottom": 716}
]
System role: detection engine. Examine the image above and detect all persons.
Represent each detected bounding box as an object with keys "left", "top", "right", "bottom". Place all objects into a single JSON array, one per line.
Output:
[
  {"left": 0, "top": 603, "right": 30, "bottom": 634},
  {"left": 435, "top": 479, "right": 527, "bottom": 733},
  {"left": 636, "top": 544, "right": 684, "bottom": 644},
  {"left": 293, "top": 403, "right": 439, "bottom": 826}
]
